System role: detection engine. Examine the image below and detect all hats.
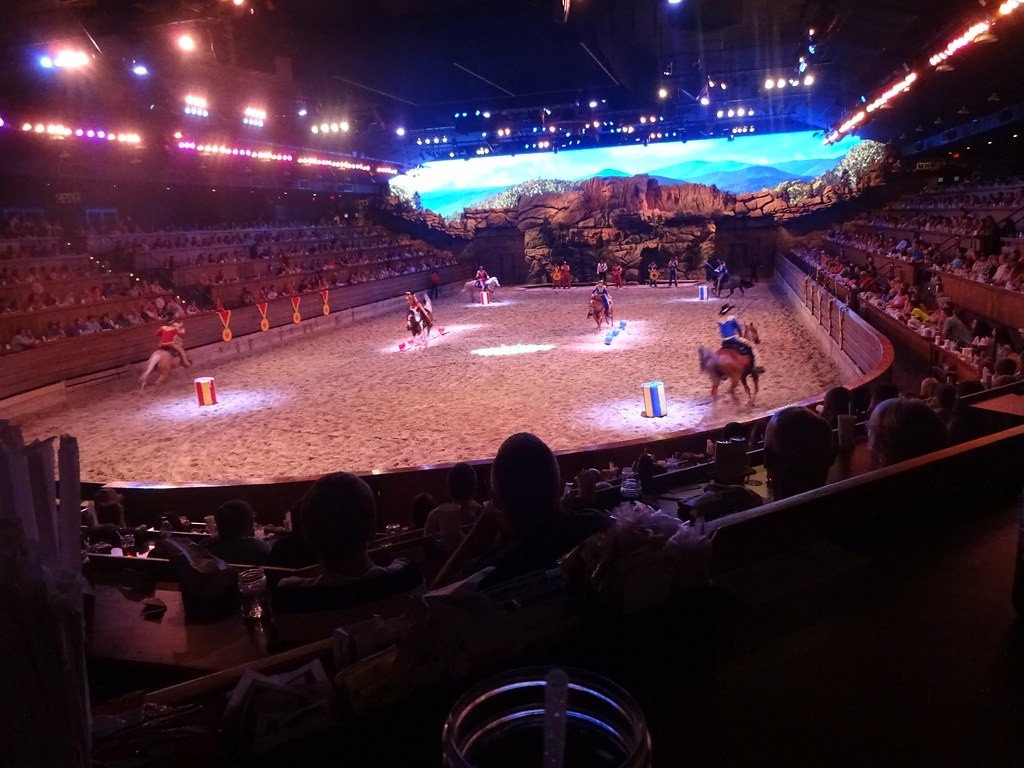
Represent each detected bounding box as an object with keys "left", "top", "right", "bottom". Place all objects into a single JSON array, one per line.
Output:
[{"left": 718, "top": 304, "right": 735, "bottom": 315}]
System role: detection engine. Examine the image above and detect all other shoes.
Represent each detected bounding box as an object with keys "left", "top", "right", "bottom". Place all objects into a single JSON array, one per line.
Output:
[{"left": 752, "top": 366, "right": 765, "bottom": 373}]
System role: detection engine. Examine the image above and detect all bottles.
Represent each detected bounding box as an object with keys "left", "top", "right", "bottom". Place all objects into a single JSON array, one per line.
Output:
[
  {"left": 667, "top": 459, "right": 679, "bottom": 473},
  {"left": 120, "top": 537, "right": 136, "bottom": 557},
  {"left": 239, "top": 569, "right": 268, "bottom": 622},
  {"left": 621, "top": 473, "right": 641, "bottom": 502},
  {"left": 442, "top": 666, "right": 653, "bottom": 768}
]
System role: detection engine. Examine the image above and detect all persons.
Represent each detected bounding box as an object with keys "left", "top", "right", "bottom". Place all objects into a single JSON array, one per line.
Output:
[
  {"left": 99, "top": 381, "right": 990, "bottom": 654},
  {"left": 668, "top": 257, "right": 678, "bottom": 287},
  {"left": 648, "top": 260, "right": 657, "bottom": 286},
  {"left": 715, "top": 259, "right": 727, "bottom": 297},
  {"left": 405, "top": 290, "right": 433, "bottom": 327},
  {"left": 476, "top": 266, "right": 489, "bottom": 292},
  {"left": 717, "top": 306, "right": 766, "bottom": 380},
  {"left": 0, "top": 205, "right": 457, "bottom": 353},
  {"left": 597, "top": 259, "right": 608, "bottom": 286},
  {"left": 155, "top": 319, "right": 192, "bottom": 367},
  {"left": 551, "top": 260, "right": 570, "bottom": 289},
  {"left": 430, "top": 272, "right": 440, "bottom": 299},
  {"left": 612, "top": 263, "right": 623, "bottom": 289},
  {"left": 587, "top": 280, "right": 610, "bottom": 325},
  {"left": 786, "top": 172, "right": 1024, "bottom": 393}
]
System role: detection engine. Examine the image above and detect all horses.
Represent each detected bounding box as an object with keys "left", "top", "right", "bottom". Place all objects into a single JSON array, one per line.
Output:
[
  {"left": 587, "top": 295, "right": 616, "bottom": 335},
  {"left": 461, "top": 277, "right": 501, "bottom": 302},
  {"left": 406, "top": 288, "right": 435, "bottom": 342},
  {"left": 698, "top": 321, "right": 765, "bottom": 419},
  {"left": 704, "top": 262, "right": 756, "bottom": 298},
  {"left": 137, "top": 322, "right": 193, "bottom": 399}
]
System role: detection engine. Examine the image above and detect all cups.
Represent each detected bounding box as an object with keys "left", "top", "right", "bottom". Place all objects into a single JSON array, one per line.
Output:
[
  {"left": 962, "top": 348, "right": 970, "bottom": 358},
  {"left": 838, "top": 415, "right": 857, "bottom": 448},
  {"left": 945, "top": 340, "right": 949, "bottom": 349},
  {"left": 896, "top": 309, "right": 900, "bottom": 319},
  {"left": 386, "top": 524, "right": 400, "bottom": 537},
  {"left": 908, "top": 316, "right": 930, "bottom": 335},
  {"left": 874, "top": 300, "right": 878, "bottom": 306},
  {"left": 936, "top": 336, "right": 940, "bottom": 344},
  {"left": 204, "top": 516, "right": 215, "bottom": 533}
]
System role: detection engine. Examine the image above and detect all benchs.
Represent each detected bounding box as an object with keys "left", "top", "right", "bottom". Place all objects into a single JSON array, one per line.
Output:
[{"left": 1, "top": 181, "right": 1024, "bottom": 768}]
